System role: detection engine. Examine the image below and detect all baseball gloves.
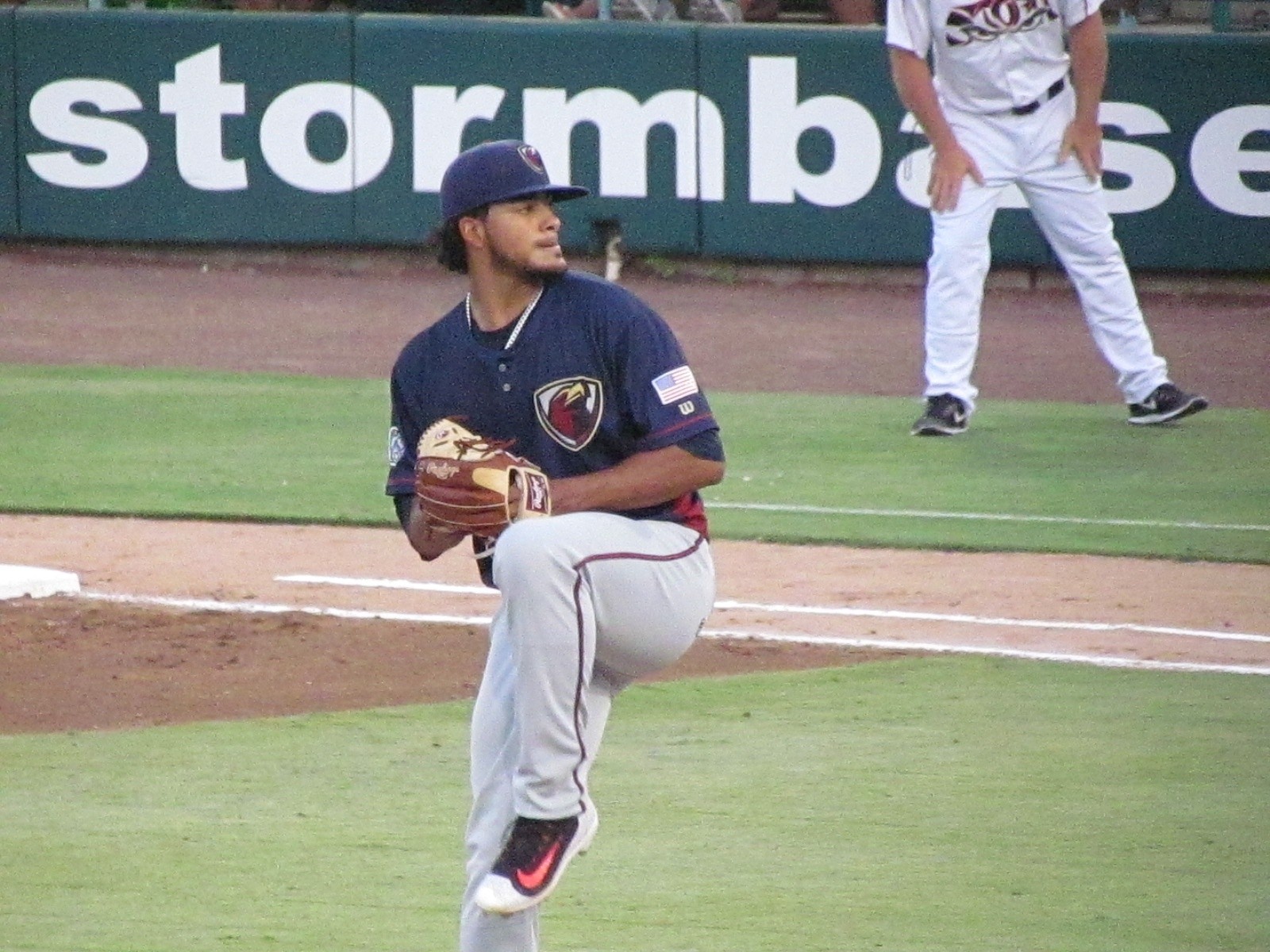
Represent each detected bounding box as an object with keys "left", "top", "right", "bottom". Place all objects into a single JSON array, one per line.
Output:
[{"left": 412, "top": 412, "right": 555, "bottom": 562}]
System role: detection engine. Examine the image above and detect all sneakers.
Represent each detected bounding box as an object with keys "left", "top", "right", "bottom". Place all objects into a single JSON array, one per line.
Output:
[
  {"left": 1129, "top": 383, "right": 1208, "bottom": 424},
  {"left": 475, "top": 812, "right": 596, "bottom": 916},
  {"left": 909, "top": 392, "right": 969, "bottom": 436}
]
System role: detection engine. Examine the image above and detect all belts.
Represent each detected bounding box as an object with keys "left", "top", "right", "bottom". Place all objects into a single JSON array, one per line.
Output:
[{"left": 1011, "top": 79, "right": 1064, "bottom": 116}]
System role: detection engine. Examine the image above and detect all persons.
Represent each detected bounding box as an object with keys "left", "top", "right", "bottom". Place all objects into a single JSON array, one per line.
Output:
[
  {"left": 523, "top": 0, "right": 881, "bottom": 24},
  {"left": 883, "top": 0, "right": 1270, "bottom": 438},
  {"left": 385, "top": 138, "right": 725, "bottom": 952}
]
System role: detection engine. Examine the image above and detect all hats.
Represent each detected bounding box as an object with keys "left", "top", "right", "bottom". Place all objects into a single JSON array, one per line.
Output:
[{"left": 441, "top": 139, "right": 588, "bottom": 222}]
